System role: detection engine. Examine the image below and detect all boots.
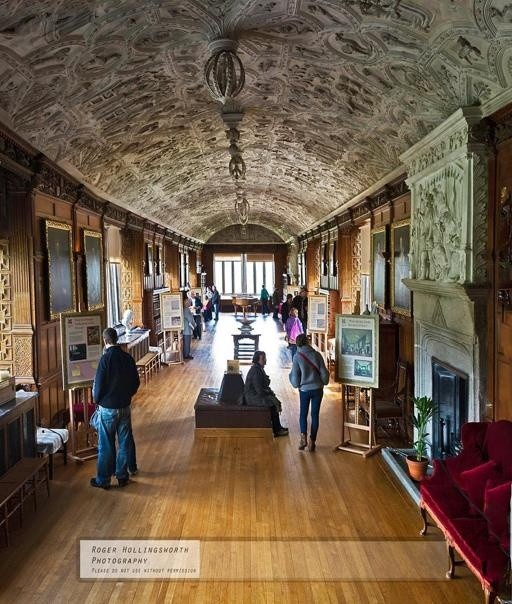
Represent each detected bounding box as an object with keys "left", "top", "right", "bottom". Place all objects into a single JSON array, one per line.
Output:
[
  {"left": 307, "top": 436, "right": 315, "bottom": 452},
  {"left": 299, "top": 433, "right": 307, "bottom": 450}
]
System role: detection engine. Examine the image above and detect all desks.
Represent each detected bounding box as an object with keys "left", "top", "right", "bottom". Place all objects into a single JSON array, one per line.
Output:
[{"left": 231, "top": 295, "right": 259, "bottom": 318}]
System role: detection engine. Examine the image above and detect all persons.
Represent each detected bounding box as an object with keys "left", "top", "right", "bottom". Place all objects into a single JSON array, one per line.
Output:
[
  {"left": 289, "top": 333, "right": 330, "bottom": 451},
  {"left": 90, "top": 327, "right": 140, "bottom": 489},
  {"left": 243, "top": 350, "right": 289, "bottom": 437},
  {"left": 272, "top": 286, "right": 308, "bottom": 361},
  {"left": 260, "top": 284, "right": 271, "bottom": 314},
  {"left": 184, "top": 285, "right": 220, "bottom": 358},
  {"left": 102, "top": 345, "right": 138, "bottom": 476}
]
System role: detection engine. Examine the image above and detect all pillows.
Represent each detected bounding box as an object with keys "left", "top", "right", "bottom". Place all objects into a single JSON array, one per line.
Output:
[{"left": 441, "top": 445, "right": 510, "bottom": 549}]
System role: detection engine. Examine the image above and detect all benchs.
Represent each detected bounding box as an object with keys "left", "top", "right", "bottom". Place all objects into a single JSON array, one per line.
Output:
[
  {"left": 134, "top": 352, "right": 160, "bottom": 386},
  {"left": 194, "top": 386, "right": 276, "bottom": 442},
  {"left": 0, "top": 457, "right": 52, "bottom": 547}
]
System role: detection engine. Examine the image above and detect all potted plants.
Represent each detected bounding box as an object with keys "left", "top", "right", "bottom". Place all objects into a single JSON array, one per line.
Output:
[{"left": 405, "top": 394, "right": 439, "bottom": 483}]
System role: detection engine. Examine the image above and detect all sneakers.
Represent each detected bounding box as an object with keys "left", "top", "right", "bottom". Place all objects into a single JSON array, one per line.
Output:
[
  {"left": 91, "top": 478, "right": 110, "bottom": 490},
  {"left": 184, "top": 355, "right": 193, "bottom": 359},
  {"left": 274, "top": 426, "right": 288, "bottom": 436},
  {"left": 118, "top": 478, "right": 128, "bottom": 487},
  {"left": 127, "top": 468, "right": 137, "bottom": 475},
  {"left": 192, "top": 335, "right": 201, "bottom": 339}
]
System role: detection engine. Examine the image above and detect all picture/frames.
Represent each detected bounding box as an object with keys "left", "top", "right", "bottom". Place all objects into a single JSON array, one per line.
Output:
[
  {"left": 60, "top": 312, "right": 108, "bottom": 391},
  {"left": 39, "top": 215, "right": 108, "bottom": 323},
  {"left": 334, "top": 313, "right": 380, "bottom": 389},
  {"left": 144, "top": 242, "right": 163, "bottom": 277},
  {"left": 159, "top": 292, "right": 185, "bottom": 332},
  {"left": 318, "top": 241, "right": 337, "bottom": 276},
  {"left": 306, "top": 294, "right": 328, "bottom": 334},
  {"left": 369, "top": 217, "right": 416, "bottom": 318}
]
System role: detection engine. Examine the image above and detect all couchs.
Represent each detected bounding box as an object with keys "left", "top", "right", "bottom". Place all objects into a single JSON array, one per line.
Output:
[{"left": 417, "top": 418, "right": 512, "bottom": 604}]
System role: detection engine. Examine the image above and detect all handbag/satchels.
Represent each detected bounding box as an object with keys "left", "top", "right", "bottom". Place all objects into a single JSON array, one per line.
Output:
[
  {"left": 290, "top": 321, "right": 301, "bottom": 341},
  {"left": 90, "top": 409, "right": 99, "bottom": 432}
]
